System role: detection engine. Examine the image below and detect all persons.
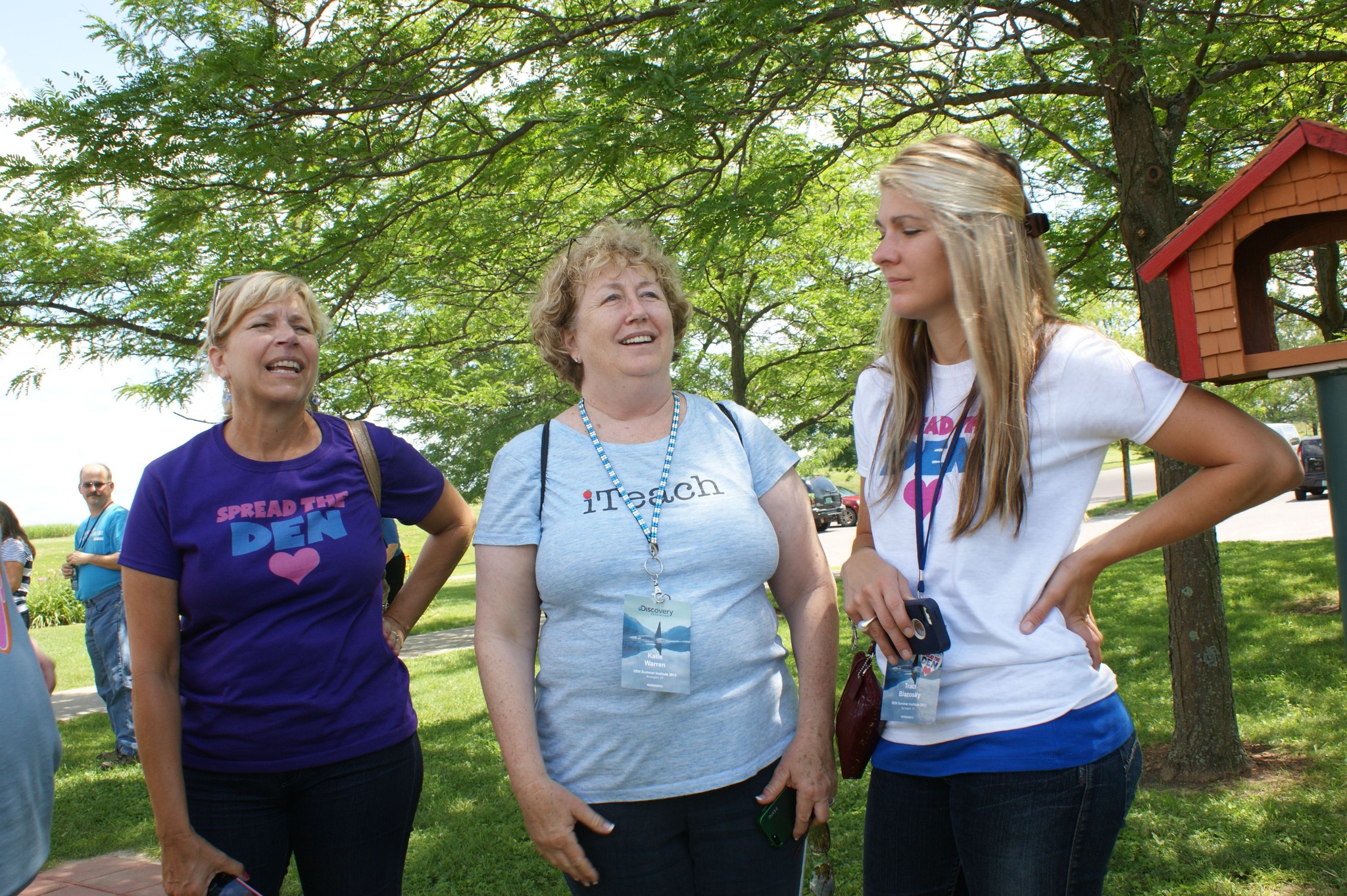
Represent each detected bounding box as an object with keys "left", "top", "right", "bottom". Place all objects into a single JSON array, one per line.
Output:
[
  {"left": 839, "top": 137, "right": 1306, "bottom": 896},
  {"left": 117, "top": 269, "right": 476, "bottom": 895},
  {"left": 381, "top": 517, "right": 407, "bottom": 608},
  {"left": 0, "top": 499, "right": 36, "bottom": 632},
  {"left": 471, "top": 221, "right": 843, "bottom": 895},
  {"left": 3, "top": 560, "right": 61, "bottom": 896},
  {"left": 61, "top": 461, "right": 141, "bottom": 772}
]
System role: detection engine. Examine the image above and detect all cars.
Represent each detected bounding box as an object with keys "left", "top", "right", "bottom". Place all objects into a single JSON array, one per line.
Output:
[{"left": 831, "top": 485, "right": 861, "bottom": 527}]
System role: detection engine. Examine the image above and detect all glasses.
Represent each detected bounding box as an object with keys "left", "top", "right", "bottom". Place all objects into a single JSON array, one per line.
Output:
[
  {"left": 80, "top": 482, "right": 111, "bottom": 488},
  {"left": 793, "top": 811, "right": 835, "bottom": 896}
]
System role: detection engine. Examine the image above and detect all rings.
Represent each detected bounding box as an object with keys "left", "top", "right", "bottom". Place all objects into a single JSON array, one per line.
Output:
[
  {"left": 828, "top": 797, "right": 836, "bottom": 807},
  {"left": 391, "top": 630, "right": 400, "bottom": 642},
  {"left": 1083, "top": 613, "right": 1090, "bottom": 623},
  {"left": 858, "top": 614, "right": 878, "bottom": 634}
]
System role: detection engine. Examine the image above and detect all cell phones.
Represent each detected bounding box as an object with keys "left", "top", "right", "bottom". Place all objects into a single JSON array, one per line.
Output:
[
  {"left": 205, "top": 871, "right": 265, "bottom": 895},
  {"left": 886, "top": 597, "right": 951, "bottom": 656},
  {"left": 757, "top": 786, "right": 798, "bottom": 850}
]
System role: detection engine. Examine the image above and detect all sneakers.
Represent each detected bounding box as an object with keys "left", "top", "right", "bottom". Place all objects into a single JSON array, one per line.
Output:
[
  {"left": 96, "top": 750, "right": 117, "bottom": 760},
  {"left": 101, "top": 753, "right": 141, "bottom": 770}
]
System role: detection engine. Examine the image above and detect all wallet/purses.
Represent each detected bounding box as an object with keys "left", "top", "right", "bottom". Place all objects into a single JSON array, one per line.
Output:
[{"left": 835, "top": 640, "right": 887, "bottom": 779}]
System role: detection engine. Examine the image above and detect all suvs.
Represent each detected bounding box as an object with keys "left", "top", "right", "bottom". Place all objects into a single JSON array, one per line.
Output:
[
  {"left": 1296, "top": 435, "right": 1329, "bottom": 500},
  {"left": 798, "top": 475, "right": 844, "bottom": 532}
]
